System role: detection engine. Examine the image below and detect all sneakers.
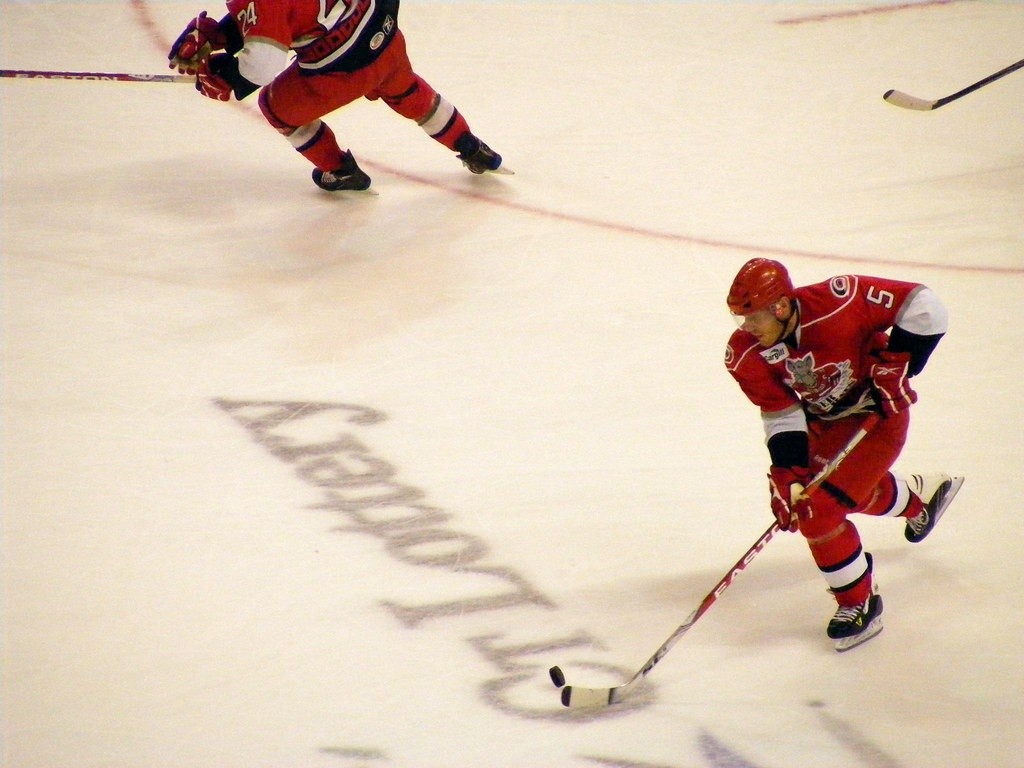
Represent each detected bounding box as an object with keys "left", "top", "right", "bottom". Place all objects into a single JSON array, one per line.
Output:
[
  {"left": 825, "top": 552, "right": 884, "bottom": 653},
  {"left": 456, "top": 135, "right": 515, "bottom": 176},
  {"left": 312, "top": 147, "right": 380, "bottom": 197},
  {"left": 904, "top": 472, "right": 965, "bottom": 543}
]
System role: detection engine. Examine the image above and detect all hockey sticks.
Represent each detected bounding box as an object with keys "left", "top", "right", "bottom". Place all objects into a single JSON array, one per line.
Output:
[
  {"left": 881, "top": 58, "right": 1024, "bottom": 113},
  {"left": 559, "top": 411, "right": 883, "bottom": 710},
  {"left": 0, "top": 68, "right": 204, "bottom": 85}
]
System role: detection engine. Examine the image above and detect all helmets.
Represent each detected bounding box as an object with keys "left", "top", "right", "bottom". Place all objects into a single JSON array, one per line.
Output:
[{"left": 726, "top": 258, "right": 796, "bottom": 332}]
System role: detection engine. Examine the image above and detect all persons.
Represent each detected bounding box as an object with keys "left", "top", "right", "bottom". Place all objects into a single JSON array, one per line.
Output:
[
  {"left": 725, "top": 258, "right": 952, "bottom": 639},
  {"left": 169, "top": 0, "right": 502, "bottom": 192}
]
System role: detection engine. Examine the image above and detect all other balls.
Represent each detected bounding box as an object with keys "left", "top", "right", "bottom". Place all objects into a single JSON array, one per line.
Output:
[{"left": 548, "top": 665, "right": 566, "bottom": 688}]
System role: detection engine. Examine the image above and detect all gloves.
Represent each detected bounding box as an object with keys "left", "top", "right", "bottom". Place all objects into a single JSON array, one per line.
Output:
[
  {"left": 766, "top": 464, "right": 814, "bottom": 533},
  {"left": 866, "top": 350, "right": 918, "bottom": 421},
  {"left": 195, "top": 52, "right": 236, "bottom": 102},
  {"left": 167, "top": 11, "right": 226, "bottom": 75}
]
